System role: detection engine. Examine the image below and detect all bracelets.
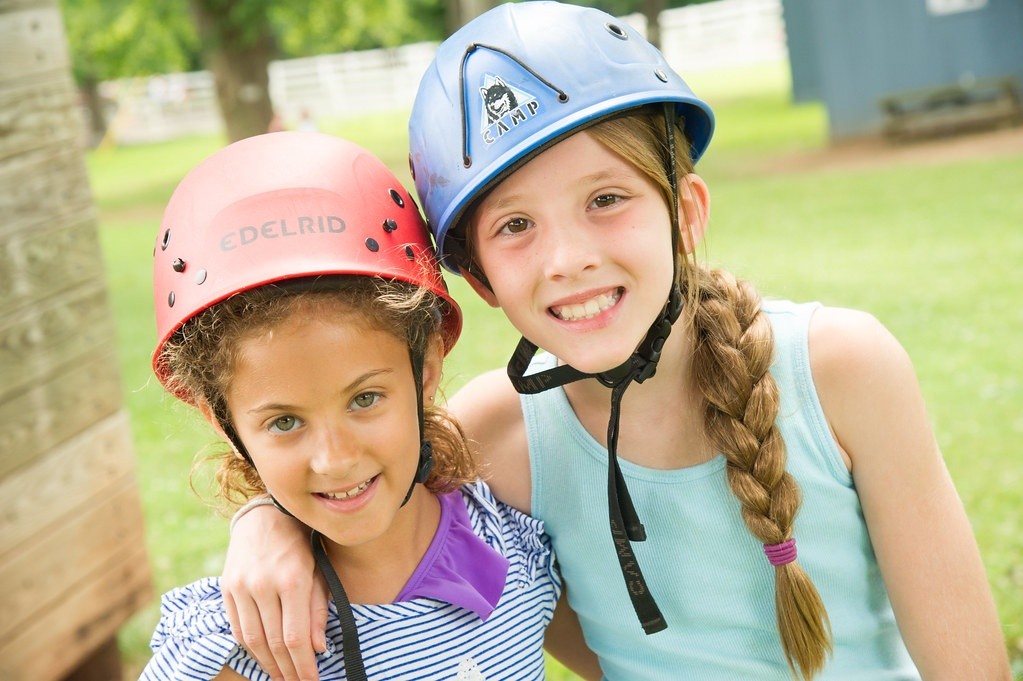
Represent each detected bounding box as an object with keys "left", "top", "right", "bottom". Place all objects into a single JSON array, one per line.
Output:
[{"left": 230, "top": 497, "right": 273, "bottom": 533}]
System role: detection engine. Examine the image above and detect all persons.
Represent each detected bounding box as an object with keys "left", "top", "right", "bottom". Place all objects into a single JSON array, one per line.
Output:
[
  {"left": 220, "top": 0, "right": 1015, "bottom": 681},
  {"left": 134, "top": 128, "right": 604, "bottom": 681}
]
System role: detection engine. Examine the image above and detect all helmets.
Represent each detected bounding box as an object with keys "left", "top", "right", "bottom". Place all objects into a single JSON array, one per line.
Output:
[
  {"left": 407, "top": 0, "right": 711, "bottom": 275},
  {"left": 151, "top": 130, "right": 462, "bottom": 407}
]
